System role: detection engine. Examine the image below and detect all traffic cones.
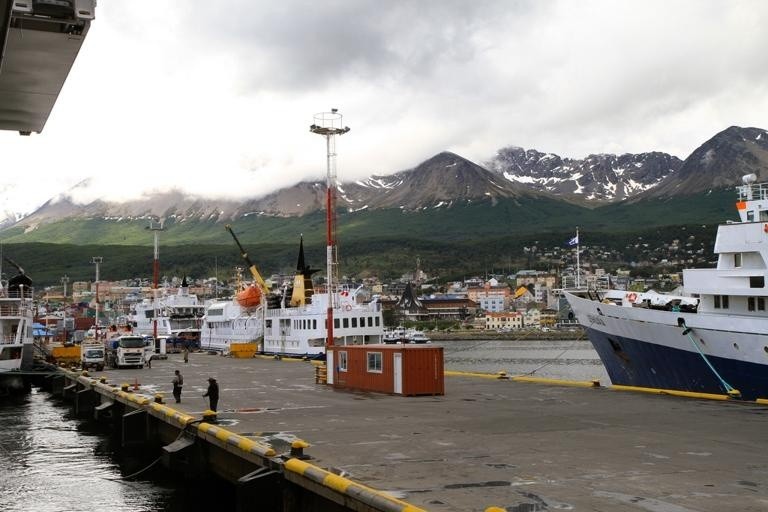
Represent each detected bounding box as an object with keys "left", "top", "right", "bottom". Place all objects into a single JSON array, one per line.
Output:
[{"left": 132, "top": 378, "right": 141, "bottom": 390}]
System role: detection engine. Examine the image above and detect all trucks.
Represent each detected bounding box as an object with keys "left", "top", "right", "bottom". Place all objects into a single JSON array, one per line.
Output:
[
  {"left": 104, "top": 334, "right": 155, "bottom": 368},
  {"left": 80, "top": 344, "right": 104, "bottom": 372}
]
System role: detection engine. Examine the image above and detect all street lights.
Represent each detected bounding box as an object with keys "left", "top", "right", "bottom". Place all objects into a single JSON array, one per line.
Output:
[
  {"left": 309, "top": 108, "right": 350, "bottom": 389},
  {"left": 144, "top": 216, "right": 165, "bottom": 339},
  {"left": 91, "top": 255, "right": 102, "bottom": 341},
  {"left": 43, "top": 286, "right": 51, "bottom": 335},
  {"left": 60, "top": 274, "right": 69, "bottom": 345}
]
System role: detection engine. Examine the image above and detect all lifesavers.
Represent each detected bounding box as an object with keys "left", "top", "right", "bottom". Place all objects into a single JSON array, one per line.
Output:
[
  {"left": 628, "top": 293, "right": 637, "bottom": 302},
  {"left": 346, "top": 305, "right": 352, "bottom": 311}
]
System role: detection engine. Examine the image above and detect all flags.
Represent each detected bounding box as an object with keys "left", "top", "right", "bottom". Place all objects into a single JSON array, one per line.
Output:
[{"left": 560, "top": 232, "right": 579, "bottom": 249}]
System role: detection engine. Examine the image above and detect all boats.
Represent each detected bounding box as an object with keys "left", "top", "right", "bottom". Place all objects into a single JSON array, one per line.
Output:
[
  {"left": 196, "top": 224, "right": 382, "bottom": 359},
  {"left": 122, "top": 275, "right": 203, "bottom": 351},
  {"left": 0, "top": 254, "right": 35, "bottom": 373},
  {"left": 382, "top": 323, "right": 431, "bottom": 344},
  {"left": 562, "top": 173, "right": 767, "bottom": 400}
]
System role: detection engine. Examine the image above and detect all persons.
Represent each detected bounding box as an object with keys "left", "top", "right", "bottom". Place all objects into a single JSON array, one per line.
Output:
[
  {"left": 202, "top": 378, "right": 219, "bottom": 412},
  {"left": 171, "top": 370, "right": 183, "bottom": 403},
  {"left": 183, "top": 347, "right": 188, "bottom": 363}
]
